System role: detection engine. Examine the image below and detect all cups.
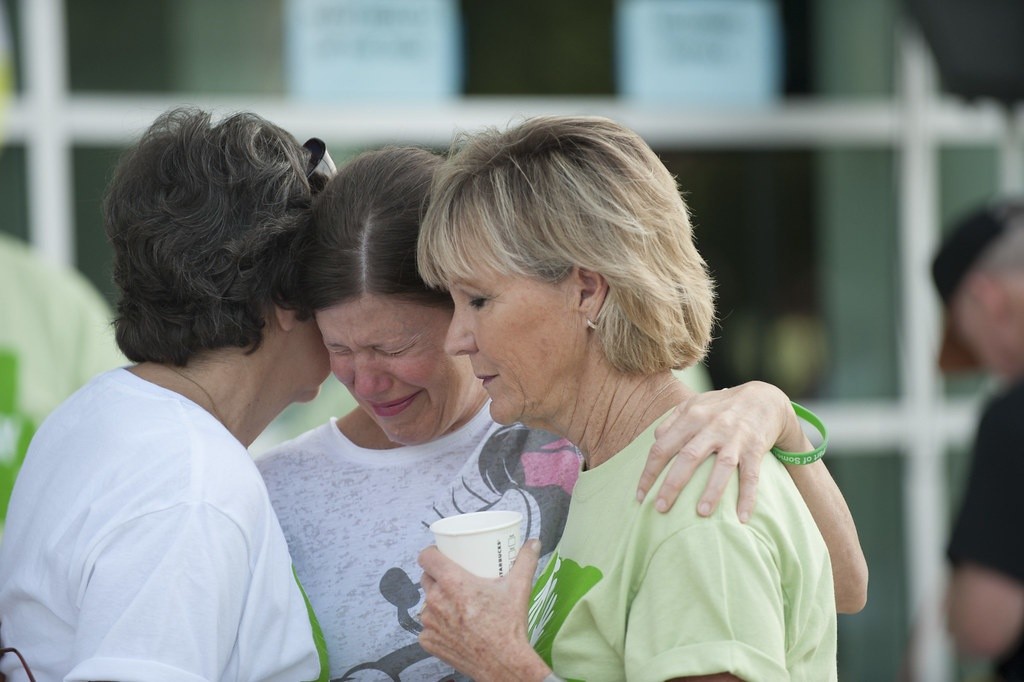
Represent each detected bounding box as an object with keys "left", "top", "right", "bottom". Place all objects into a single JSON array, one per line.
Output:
[{"left": 429, "top": 509, "right": 523, "bottom": 579}]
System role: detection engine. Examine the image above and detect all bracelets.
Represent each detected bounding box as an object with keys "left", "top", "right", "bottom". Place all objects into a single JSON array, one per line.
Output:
[{"left": 771, "top": 402, "right": 830, "bottom": 467}]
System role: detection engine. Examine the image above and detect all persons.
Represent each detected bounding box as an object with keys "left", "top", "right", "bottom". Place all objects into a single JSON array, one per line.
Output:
[
  {"left": 1, "top": 102, "right": 339, "bottom": 682},
  {"left": 931, "top": 195, "right": 1024, "bottom": 681},
  {"left": 248, "top": 147, "right": 870, "bottom": 680},
  {"left": 415, "top": 115, "right": 841, "bottom": 682}
]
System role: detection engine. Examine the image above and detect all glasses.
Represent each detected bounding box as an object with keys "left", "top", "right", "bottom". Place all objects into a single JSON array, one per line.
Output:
[{"left": 303, "top": 137, "right": 337, "bottom": 195}]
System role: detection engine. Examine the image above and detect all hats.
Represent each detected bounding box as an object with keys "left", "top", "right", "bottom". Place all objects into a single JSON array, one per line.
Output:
[{"left": 930, "top": 194, "right": 1024, "bottom": 369}]
